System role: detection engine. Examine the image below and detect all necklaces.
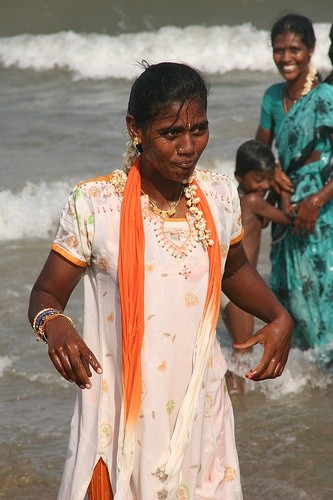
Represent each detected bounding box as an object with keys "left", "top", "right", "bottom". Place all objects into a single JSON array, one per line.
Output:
[
  {"left": 284, "top": 87, "right": 299, "bottom": 102},
  {"left": 139, "top": 184, "right": 186, "bottom": 219}
]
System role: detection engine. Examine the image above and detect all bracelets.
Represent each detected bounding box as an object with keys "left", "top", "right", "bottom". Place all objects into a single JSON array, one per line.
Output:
[{"left": 33, "top": 307, "right": 77, "bottom": 344}]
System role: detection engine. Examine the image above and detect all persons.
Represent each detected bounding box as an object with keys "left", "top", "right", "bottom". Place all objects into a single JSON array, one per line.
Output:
[
  {"left": 257, "top": 14, "right": 333, "bottom": 374},
  {"left": 218, "top": 138, "right": 293, "bottom": 393},
  {"left": 28, "top": 60, "right": 294, "bottom": 500}
]
